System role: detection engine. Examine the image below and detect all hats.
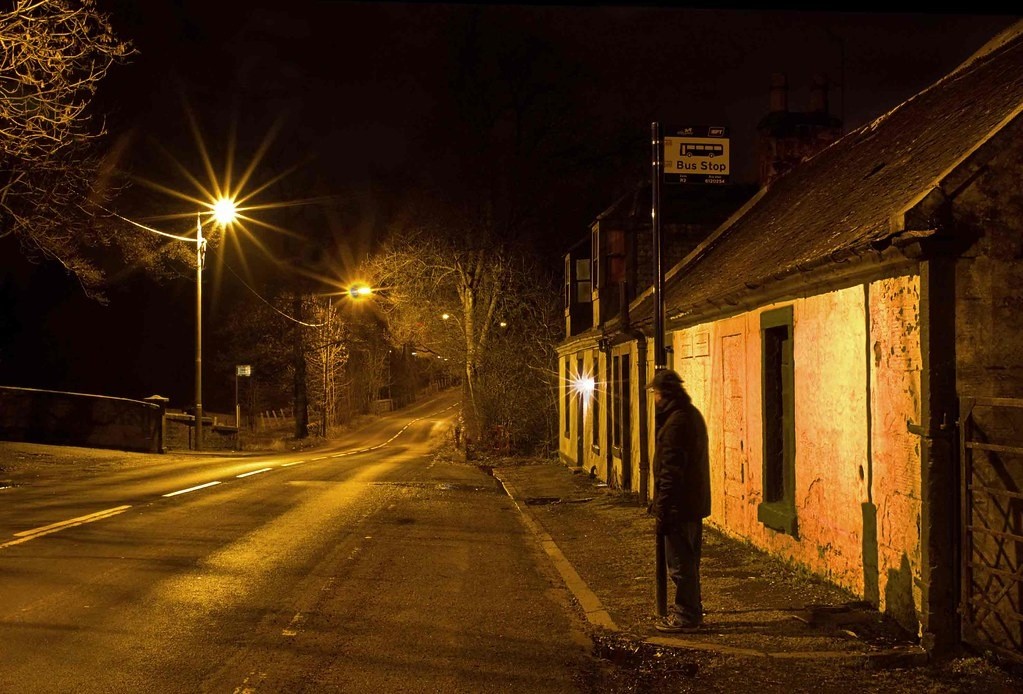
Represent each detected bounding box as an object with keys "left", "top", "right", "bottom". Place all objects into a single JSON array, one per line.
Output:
[{"left": 645, "top": 367, "right": 684, "bottom": 391}]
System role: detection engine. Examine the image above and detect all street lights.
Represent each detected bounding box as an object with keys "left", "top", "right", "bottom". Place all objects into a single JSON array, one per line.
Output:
[
  {"left": 442, "top": 311, "right": 466, "bottom": 340},
  {"left": 195, "top": 197, "right": 236, "bottom": 448}
]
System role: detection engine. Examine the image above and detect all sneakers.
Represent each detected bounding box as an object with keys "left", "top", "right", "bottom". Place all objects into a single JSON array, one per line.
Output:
[{"left": 655, "top": 610, "right": 704, "bottom": 632}]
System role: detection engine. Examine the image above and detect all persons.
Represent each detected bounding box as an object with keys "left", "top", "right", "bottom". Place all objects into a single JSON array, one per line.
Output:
[{"left": 644, "top": 370, "right": 711, "bottom": 633}]
